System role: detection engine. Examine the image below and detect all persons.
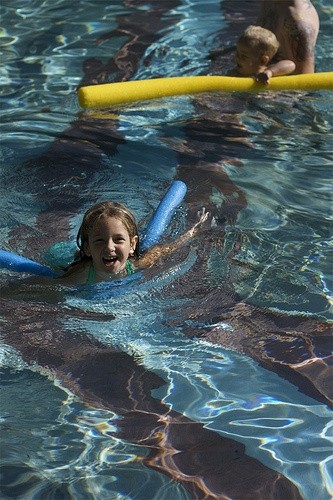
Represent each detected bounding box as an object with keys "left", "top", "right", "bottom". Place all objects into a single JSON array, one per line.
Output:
[
  {"left": 255, "top": 0, "right": 321, "bottom": 75},
  {"left": 185, "top": 24, "right": 296, "bottom": 165},
  {"left": 3, "top": 200, "right": 210, "bottom": 289}
]
